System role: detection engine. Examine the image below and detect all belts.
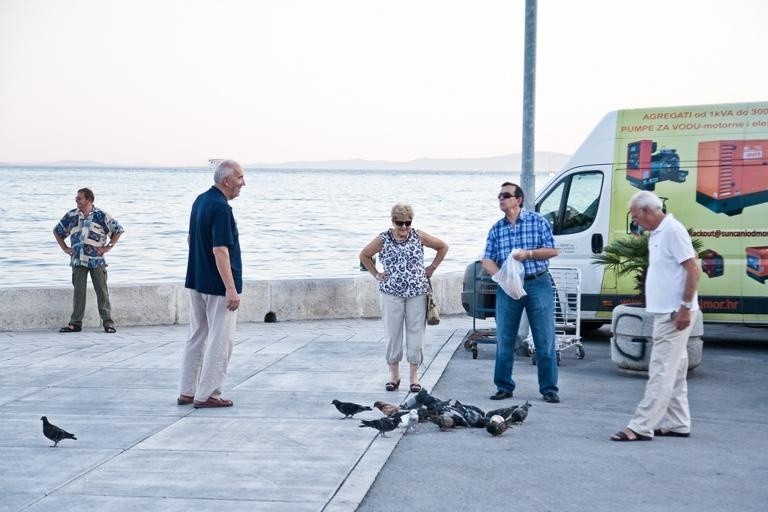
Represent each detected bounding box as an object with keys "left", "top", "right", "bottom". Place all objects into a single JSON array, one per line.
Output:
[{"left": 524, "top": 271, "right": 546, "bottom": 281}]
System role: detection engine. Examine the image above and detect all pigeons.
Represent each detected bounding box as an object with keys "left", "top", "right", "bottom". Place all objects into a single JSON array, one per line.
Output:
[
  {"left": 40, "top": 415, "right": 78, "bottom": 448},
  {"left": 332, "top": 387, "right": 532, "bottom": 437}
]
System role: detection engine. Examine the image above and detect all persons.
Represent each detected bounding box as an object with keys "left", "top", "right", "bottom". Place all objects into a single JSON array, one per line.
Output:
[
  {"left": 177, "top": 161, "right": 246, "bottom": 408},
  {"left": 610, "top": 190, "right": 702, "bottom": 442},
  {"left": 52, "top": 188, "right": 125, "bottom": 333},
  {"left": 481, "top": 181, "right": 560, "bottom": 403},
  {"left": 359, "top": 204, "right": 449, "bottom": 392}
]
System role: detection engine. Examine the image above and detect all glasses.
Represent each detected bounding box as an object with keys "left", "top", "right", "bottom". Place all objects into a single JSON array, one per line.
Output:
[
  {"left": 393, "top": 220, "right": 412, "bottom": 226},
  {"left": 498, "top": 191, "right": 516, "bottom": 199}
]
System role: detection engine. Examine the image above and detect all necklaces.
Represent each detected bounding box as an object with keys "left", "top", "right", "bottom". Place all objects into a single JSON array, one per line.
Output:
[{"left": 387, "top": 231, "right": 412, "bottom": 245}]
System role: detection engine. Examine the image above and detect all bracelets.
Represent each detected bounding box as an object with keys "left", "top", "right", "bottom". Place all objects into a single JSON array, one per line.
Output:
[
  {"left": 376, "top": 273, "right": 380, "bottom": 280},
  {"left": 680, "top": 300, "right": 693, "bottom": 309},
  {"left": 430, "top": 264, "right": 437, "bottom": 270},
  {"left": 108, "top": 242, "right": 114, "bottom": 248},
  {"left": 528, "top": 250, "right": 533, "bottom": 260}
]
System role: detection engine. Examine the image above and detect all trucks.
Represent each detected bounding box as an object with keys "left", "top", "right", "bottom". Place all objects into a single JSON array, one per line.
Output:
[{"left": 457, "top": 102, "right": 767, "bottom": 326}]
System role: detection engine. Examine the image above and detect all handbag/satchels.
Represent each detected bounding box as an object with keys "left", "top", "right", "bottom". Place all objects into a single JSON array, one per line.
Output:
[{"left": 427, "top": 278, "right": 440, "bottom": 324}]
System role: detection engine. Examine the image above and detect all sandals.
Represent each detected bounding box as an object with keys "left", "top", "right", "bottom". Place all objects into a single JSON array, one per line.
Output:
[
  {"left": 410, "top": 384, "right": 421, "bottom": 392},
  {"left": 610, "top": 428, "right": 652, "bottom": 441},
  {"left": 59, "top": 324, "right": 81, "bottom": 332},
  {"left": 654, "top": 428, "right": 690, "bottom": 437},
  {"left": 105, "top": 326, "right": 116, "bottom": 333},
  {"left": 386, "top": 379, "right": 400, "bottom": 391}
]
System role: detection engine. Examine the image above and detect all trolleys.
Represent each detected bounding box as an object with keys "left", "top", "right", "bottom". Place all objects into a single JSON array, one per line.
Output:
[{"left": 462, "top": 258, "right": 586, "bottom": 360}]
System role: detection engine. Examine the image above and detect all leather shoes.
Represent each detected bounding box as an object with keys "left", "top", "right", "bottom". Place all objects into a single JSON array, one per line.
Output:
[
  {"left": 543, "top": 392, "right": 560, "bottom": 403},
  {"left": 490, "top": 390, "right": 513, "bottom": 399},
  {"left": 192, "top": 397, "right": 233, "bottom": 408},
  {"left": 177, "top": 394, "right": 194, "bottom": 404}
]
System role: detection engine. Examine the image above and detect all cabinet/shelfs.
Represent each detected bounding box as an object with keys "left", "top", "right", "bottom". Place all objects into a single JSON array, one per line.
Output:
[
  {"left": 530, "top": 267, "right": 584, "bottom": 365},
  {"left": 466, "top": 260, "right": 500, "bottom": 359}
]
